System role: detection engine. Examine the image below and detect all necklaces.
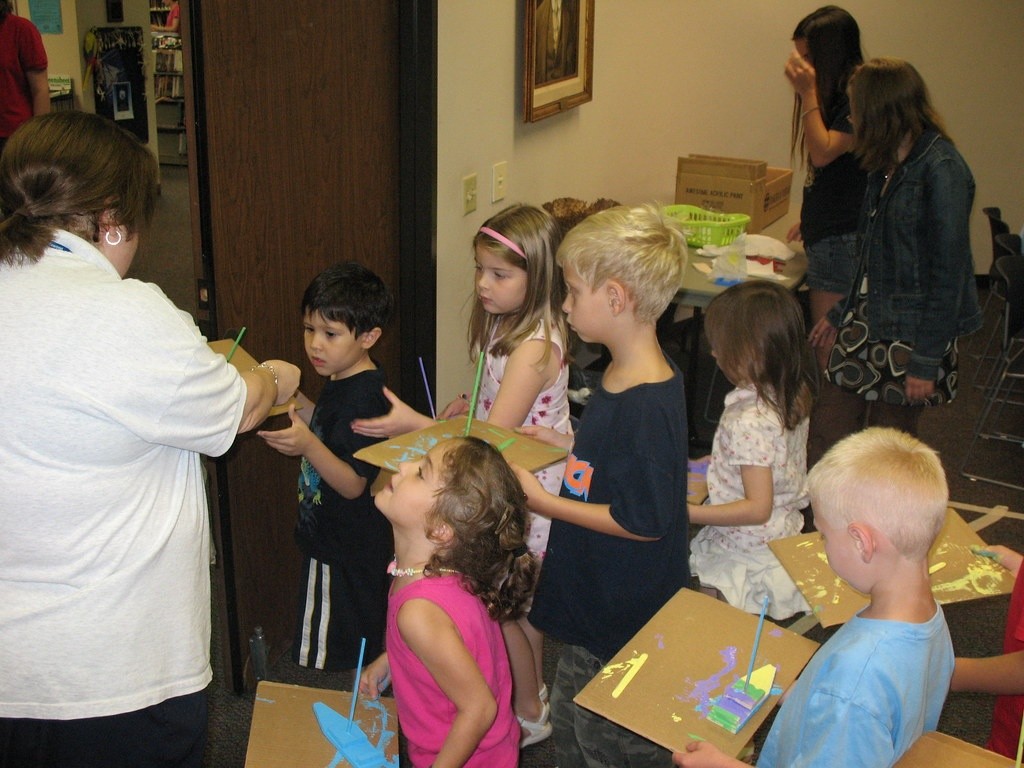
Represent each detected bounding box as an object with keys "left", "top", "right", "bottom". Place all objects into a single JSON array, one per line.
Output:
[{"left": 386, "top": 554, "right": 462, "bottom": 575}]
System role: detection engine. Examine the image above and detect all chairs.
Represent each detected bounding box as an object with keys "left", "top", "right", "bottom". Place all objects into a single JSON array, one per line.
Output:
[{"left": 958, "top": 207, "right": 1024, "bottom": 492}]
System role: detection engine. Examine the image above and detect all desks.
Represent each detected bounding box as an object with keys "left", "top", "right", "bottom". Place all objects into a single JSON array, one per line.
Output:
[{"left": 656, "top": 202, "right": 809, "bottom": 442}]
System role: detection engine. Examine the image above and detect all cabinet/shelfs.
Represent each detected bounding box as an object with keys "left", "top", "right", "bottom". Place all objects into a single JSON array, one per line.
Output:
[{"left": 150, "top": 0, "right": 188, "bottom": 166}]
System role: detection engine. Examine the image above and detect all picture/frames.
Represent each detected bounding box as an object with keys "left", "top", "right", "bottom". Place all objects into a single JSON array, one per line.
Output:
[
  {"left": 106, "top": 0, "right": 124, "bottom": 22},
  {"left": 523, "top": 0, "right": 595, "bottom": 123}
]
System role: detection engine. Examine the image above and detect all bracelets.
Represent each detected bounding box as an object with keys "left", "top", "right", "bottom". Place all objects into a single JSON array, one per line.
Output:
[
  {"left": 251, "top": 362, "right": 279, "bottom": 407},
  {"left": 801, "top": 107, "right": 819, "bottom": 118},
  {"left": 457, "top": 391, "right": 472, "bottom": 414}
]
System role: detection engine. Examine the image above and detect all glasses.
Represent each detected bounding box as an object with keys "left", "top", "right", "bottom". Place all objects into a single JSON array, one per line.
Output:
[{"left": 846, "top": 114, "right": 853, "bottom": 122}]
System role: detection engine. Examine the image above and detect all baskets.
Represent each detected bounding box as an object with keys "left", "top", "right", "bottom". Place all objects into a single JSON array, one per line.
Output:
[{"left": 663, "top": 204, "right": 751, "bottom": 248}]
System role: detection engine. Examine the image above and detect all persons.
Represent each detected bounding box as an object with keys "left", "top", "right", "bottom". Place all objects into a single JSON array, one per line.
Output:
[
  {"left": 504, "top": 203, "right": 692, "bottom": 768},
  {"left": 0, "top": 1, "right": 51, "bottom": 143},
  {"left": 150, "top": 0, "right": 180, "bottom": 34},
  {"left": 947, "top": 545, "right": 1024, "bottom": 762},
  {"left": 358, "top": 437, "right": 536, "bottom": 768},
  {"left": 254, "top": 258, "right": 394, "bottom": 671},
  {"left": 805, "top": 60, "right": 986, "bottom": 533},
  {"left": 684, "top": 279, "right": 813, "bottom": 623},
  {"left": 781, "top": 4, "right": 868, "bottom": 374},
  {"left": 0, "top": 109, "right": 301, "bottom": 768},
  {"left": 349, "top": 204, "right": 575, "bottom": 615},
  {"left": 668, "top": 427, "right": 955, "bottom": 768}
]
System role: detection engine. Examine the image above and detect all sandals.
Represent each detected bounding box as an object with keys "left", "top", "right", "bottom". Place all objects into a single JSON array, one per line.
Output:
[
  {"left": 516, "top": 703, "right": 553, "bottom": 748},
  {"left": 538, "top": 684, "right": 549, "bottom": 703}
]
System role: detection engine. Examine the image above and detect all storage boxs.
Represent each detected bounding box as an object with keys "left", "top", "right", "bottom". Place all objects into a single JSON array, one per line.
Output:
[{"left": 675, "top": 154, "right": 793, "bottom": 234}]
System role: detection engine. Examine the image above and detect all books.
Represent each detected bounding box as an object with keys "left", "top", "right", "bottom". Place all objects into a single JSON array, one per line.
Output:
[
  {"left": 151, "top": 0, "right": 165, "bottom": 9},
  {"left": 154, "top": 75, "right": 184, "bottom": 97},
  {"left": 151, "top": 12, "right": 168, "bottom": 27},
  {"left": 156, "top": 51, "right": 184, "bottom": 72}
]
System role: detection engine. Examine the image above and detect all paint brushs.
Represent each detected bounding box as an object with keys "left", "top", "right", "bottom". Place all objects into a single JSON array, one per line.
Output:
[
  {"left": 226, "top": 324, "right": 247, "bottom": 363},
  {"left": 744, "top": 598, "right": 771, "bottom": 689},
  {"left": 345, "top": 638, "right": 370, "bottom": 726},
  {"left": 418, "top": 356, "right": 440, "bottom": 419},
  {"left": 467, "top": 352, "right": 483, "bottom": 434}
]
